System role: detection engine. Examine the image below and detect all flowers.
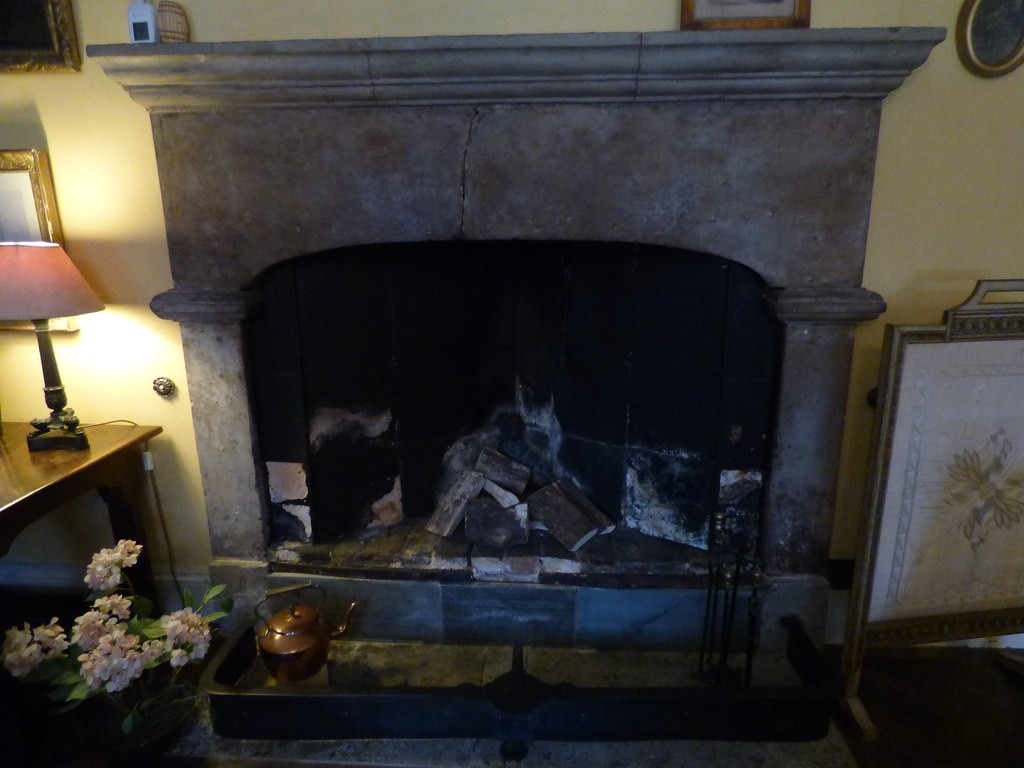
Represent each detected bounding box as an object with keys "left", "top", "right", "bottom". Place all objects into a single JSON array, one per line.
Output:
[{"left": 0, "top": 538, "right": 234, "bottom": 735}]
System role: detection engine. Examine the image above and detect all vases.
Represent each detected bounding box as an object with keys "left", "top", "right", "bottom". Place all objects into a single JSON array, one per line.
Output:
[{"left": 89, "top": 678, "right": 206, "bottom": 768}]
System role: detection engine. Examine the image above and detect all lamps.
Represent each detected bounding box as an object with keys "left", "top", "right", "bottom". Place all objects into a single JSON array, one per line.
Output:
[{"left": 0, "top": 240, "right": 106, "bottom": 453}]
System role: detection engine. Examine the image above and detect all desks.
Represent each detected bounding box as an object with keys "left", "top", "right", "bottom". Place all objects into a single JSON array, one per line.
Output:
[{"left": 0, "top": 421, "right": 163, "bottom": 690}]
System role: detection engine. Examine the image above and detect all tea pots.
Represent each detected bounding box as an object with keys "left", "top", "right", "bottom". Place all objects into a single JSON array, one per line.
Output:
[{"left": 253, "top": 581, "right": 360, "bottom": 684}]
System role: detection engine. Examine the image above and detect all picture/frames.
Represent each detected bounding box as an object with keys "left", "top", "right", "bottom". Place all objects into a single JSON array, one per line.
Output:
[
  {"left": 0, "top": 147, "right": 81, "bottom": 334},
  {"left": 955, "top": 0, "right": 1024, "bottom": 78},
  {"left": 854, "top": 278, "right": 1024, "bottom": 651},
  {"left": 0, "top": 0, "right": 83, "bottom": 76},
  {"left": 681, "top": 0, "right": 812, "bottom": 32}
]
